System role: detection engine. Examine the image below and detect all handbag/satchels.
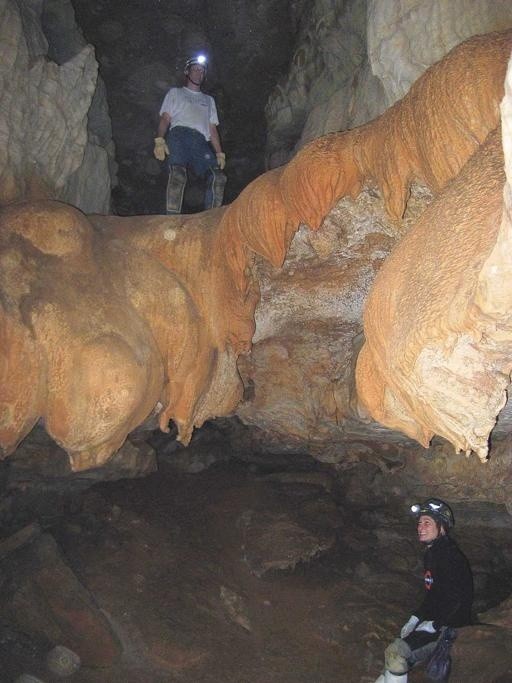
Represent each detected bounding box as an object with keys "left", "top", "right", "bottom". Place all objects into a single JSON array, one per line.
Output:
[{"left": 424, "top": 626, "right": 456, "bottom": 681}]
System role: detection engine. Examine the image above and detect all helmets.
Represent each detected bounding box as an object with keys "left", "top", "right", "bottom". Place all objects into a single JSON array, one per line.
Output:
[
  {"left": 418, "top": 498, "right": 454, "bottom": 527},
  {"left": 184, "top": 56, "right": 209, "bottom": 71}
]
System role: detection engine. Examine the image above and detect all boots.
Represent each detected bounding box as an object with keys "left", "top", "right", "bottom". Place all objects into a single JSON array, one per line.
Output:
[{"left": 374, "top": 671, "right": 408, "bottom": 683}]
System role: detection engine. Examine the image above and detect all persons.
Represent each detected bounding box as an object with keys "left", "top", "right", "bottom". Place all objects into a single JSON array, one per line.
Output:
[
  {"left": 374, "top": 497, "right": 476, "bottom": 683},
  {"left": 153, "top": 56, "right": 228, "bottom": 213}
]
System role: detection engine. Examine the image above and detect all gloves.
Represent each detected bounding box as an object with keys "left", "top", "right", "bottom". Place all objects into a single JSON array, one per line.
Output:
[
  {"left": 216, "top": 152, "right": 226, "bottom": 170},
  {"left": 399, "top": 615, "right": 419, "bottom": 639},
  {"left": 416, "top": 621, "right": 436, "bottom": 634},
  {"left": 153, "top": 137, "right": 170, "bottom": 161}
]
